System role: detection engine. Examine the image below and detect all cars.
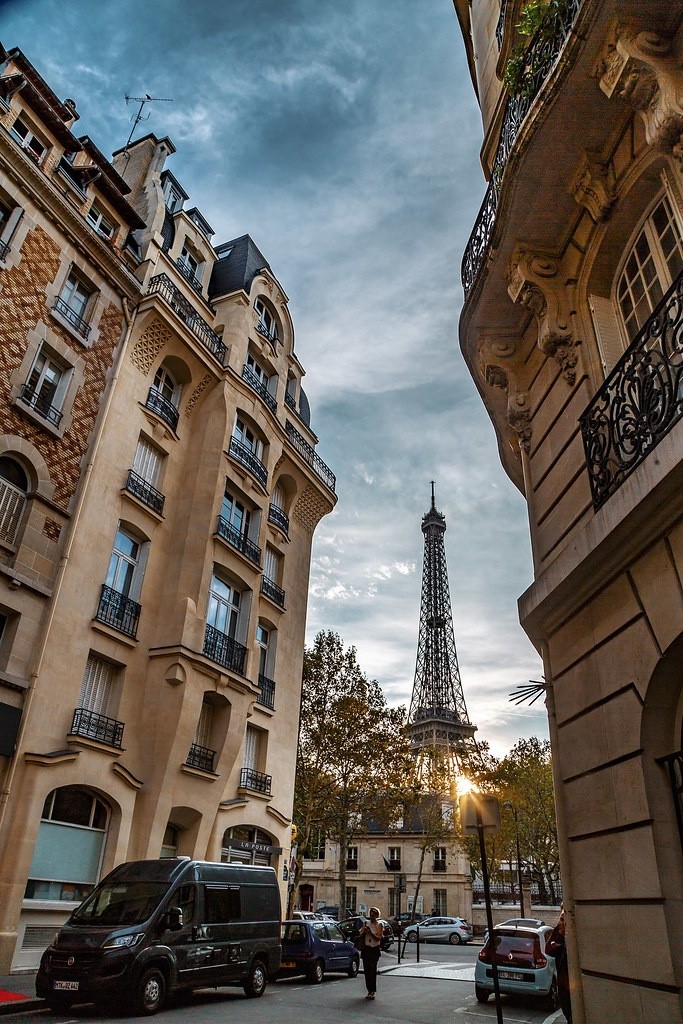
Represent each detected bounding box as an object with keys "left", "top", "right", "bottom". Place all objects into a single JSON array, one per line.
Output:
[
  {"left": 483, "top": 918, "right": 546, "bottom": 944},
  {"left": 386, "top": 911, "right": 431, "bottom": 936},
  {"left": 402, "top": 917, "right": 473, "bottom": 945},
  {"left": 336, "top": 915, "right": 395, "bottom": 951},
  {"left": 474, "top": 922, "right": 558, "bottom": 1012}
]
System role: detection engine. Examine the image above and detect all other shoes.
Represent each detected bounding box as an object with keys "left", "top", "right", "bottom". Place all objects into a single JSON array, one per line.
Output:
[{"left": 365, "top": 994, "right": 375, "bottom": 1000}]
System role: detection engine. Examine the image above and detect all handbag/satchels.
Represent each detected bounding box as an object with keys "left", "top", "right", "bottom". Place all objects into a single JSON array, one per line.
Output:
[{"left": 353, "top": 933, "right": 366, "bottom": 951}]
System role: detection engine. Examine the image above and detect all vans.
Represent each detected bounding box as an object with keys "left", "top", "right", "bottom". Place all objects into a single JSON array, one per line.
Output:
[
  {"left": 292, "top": 905, "right": 354, "bottom": 922},
  {"left": 34, "top": 854, "right": 283, "bottom": 1018},
  {"left": 279, "top": 919, "right": 361, "bottom": 985}
]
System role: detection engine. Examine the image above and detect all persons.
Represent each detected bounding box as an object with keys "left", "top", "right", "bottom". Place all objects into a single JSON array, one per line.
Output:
[
  {"left": 358, "top": 907, "right": 384, "bottom": 999},
  {"left": 430, "top": 908, "right": 437, "bottom": 917},
  {"left": 544, "top": 901, "right": 571, "bottom": 1024}
]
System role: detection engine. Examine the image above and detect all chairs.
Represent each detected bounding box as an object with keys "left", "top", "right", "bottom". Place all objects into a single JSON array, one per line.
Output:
[
  {"left": 291, "top": 930, "right": 302, "bottom": 941},
  {"left": 316, "top": 929, "right": 326, "bottom": 939}
]
